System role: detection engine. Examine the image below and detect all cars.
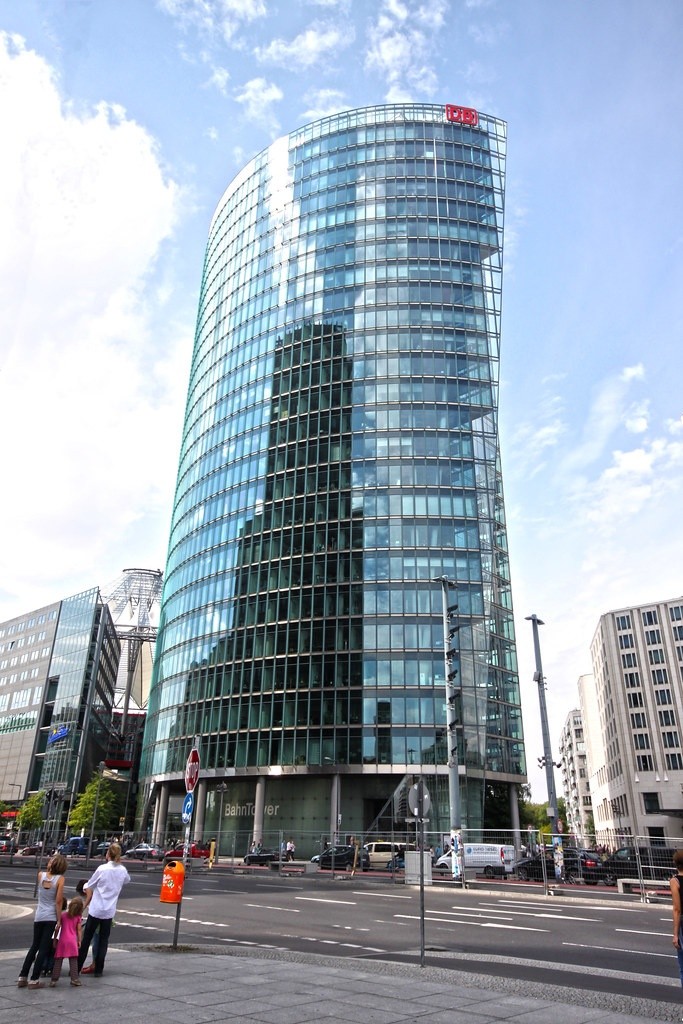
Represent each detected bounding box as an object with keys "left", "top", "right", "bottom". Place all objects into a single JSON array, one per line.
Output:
[
  {"left": 97, "top": 842, "right": 114, "bottom": 857},
  {"left": 22, "top": 841, "right": 55, "bottom": 857},
  {"left": 126, "top": 843, "right": 165, "bottom": 861},
  {"left": 244, "top": 849, "right": 290, "bottom": 866}
]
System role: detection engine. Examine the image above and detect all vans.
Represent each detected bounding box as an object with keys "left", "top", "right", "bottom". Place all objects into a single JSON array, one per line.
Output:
[
  {"left": 434, "top": 844, "right": 516, "bottom": 880},
  {"left": 362, "top": 842, "right": 417, "bottom": 867}
]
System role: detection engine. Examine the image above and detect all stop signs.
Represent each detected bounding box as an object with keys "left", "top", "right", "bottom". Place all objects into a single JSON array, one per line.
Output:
[{"left": 184, "top": 748, "right": 199, "bottom": 796}]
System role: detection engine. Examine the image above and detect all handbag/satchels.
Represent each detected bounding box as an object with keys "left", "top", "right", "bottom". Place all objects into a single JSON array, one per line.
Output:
[{"left": 49, "top": 938, "right": 59, "bottom": 952}]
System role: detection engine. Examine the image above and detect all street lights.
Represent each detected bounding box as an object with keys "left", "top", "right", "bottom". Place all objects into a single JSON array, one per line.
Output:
[
  {"left": 524, "top": 613, "right": 573, "bottom": 885},
  {"left": 9, "top": 784, "right": 22, "bottom": 840},
  {"left": 431, "top": 573, "right": 469, "bottom": 881}
]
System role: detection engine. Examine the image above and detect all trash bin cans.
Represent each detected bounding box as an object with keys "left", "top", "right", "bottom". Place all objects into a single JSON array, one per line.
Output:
[{"left": 158, "top": 860, "right": 185, "bottom": 904}]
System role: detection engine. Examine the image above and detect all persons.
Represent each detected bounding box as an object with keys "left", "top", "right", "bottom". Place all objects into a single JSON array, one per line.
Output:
[
  {"left": 250, "top": 839, "right": 264, "bottom": 854},
  {"left": 69, "top": 843, "right": 131, "bottom": 976},
  {"left": 48, "top": 897, "right": 82, "bottom": 986},
  {"left": 429, "top": 845, "right": 436, "bottom": 867},
  {"left": 594, "top": 843, "right": 616, "bottom": 861},
  {"left": 670, "top": 849, "right": 683, "bottom": 989},
  {"left": 76, "top": 879, "right": 116, "bottom": 974},
  {"left": 18, "top": 854, "right": 66, "bottom": 990},
  {"left": 394, "top": 844, "right": 405, "bottom": 869},
  {"left": 281, "top": 836, "right": 296, "bottom": 862},
  {"left": 167, "top": 837, "right": 211, "bottom": 850},
  {"left": 111, "top": 834, "right": 145, "bottom": 855},
  {"left": 40, "top": 897, "right": 67, "bottom": 977},
  {"left": 435, "top": 844, "right": 441, "bottom": 860}
]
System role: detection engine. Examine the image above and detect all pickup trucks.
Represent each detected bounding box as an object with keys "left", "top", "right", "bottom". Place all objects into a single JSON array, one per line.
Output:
[
  {"left": 164, "top": 843, "right": 210, "bottom": 859},
  {"left": 0, "top": 835, "right": 19, "bottom": 854}
]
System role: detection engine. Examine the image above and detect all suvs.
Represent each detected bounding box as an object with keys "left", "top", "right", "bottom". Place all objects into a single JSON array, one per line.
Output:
[
  {"left": 386, "top": 856, "right": 406, "bottom": 870},
  {"left": 514, "top": 846, "right": 608, "bottom": 881},
  {"left": 600, "top": 847, "right": 681, "bottom": 885},
  {"left": 311, "top": 845, "right": 376, "bottom": 872},
  {"left": 57, "top": 836, "right": 94, "bottom": 857}
]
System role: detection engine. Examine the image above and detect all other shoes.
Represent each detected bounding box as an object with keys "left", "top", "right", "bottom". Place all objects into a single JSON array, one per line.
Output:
[
  {"left": 18, "top": 978, "right": 28, "bottom": 987},
  {"left": 81, "top": 965, "right": 96, "bottom": 973},
  {"left": 70, "top": 978, "right": 83, "bottom": 986},
  {"left": 46, "top": 970, "right": 53, "bottom": 977},
  {"left": 94, "top": 973, "right": 102, "bottom": 977},
  {"left": 48, "top": 980, "right": 58, "bottom": 987},
  {"left": 40, "top": 970, "right": 46, "bottom": 977},
  {"left": 28, "top": 981, "right": 44, "bottom": 988}
]
